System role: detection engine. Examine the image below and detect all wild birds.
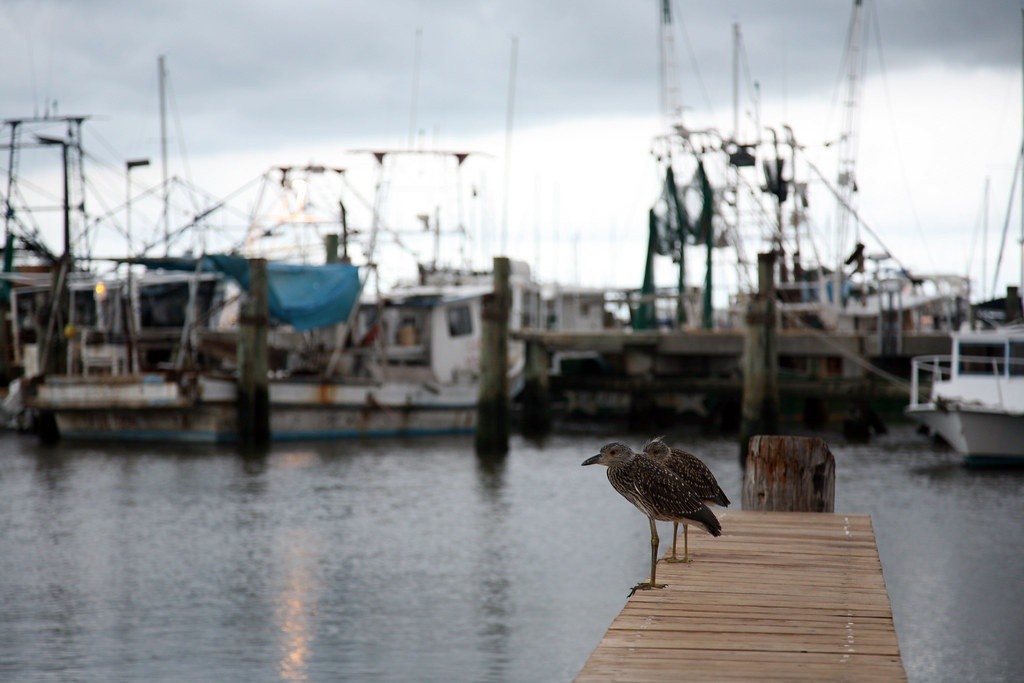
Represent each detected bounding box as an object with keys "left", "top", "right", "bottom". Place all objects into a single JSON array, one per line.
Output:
[{"left": 581, "top": 435, "right": 731, "bottom": 598}]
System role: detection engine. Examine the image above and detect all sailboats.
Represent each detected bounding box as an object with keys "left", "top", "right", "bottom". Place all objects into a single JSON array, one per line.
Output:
[{"left": 0, "top": 2, "right": 1024, "bottom": 470}]
